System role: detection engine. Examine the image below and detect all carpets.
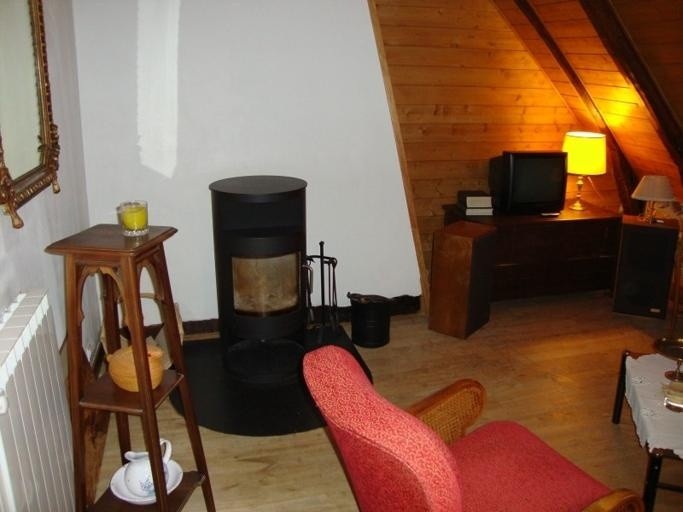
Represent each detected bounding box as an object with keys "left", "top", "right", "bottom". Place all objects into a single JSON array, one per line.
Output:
[{"left": 163, "top": 326, "right": 375, "bottom": 439}]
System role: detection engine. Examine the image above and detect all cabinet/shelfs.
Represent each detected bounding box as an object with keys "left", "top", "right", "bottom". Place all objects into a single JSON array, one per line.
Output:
[{"left": 44, "top": 224, "right": 216, "bottom": 510}]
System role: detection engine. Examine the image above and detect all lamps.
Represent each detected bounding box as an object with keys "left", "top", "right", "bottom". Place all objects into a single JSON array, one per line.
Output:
[
  {"left": 561, "top": 131, "right": 609, "bottom": 210},
  {"left": 631, "top": 175, "right": 675, "bottom": 219}
]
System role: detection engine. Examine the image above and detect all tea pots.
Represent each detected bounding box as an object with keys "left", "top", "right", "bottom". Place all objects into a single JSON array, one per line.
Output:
[{"left": 124, "top": 438, "right": 174, "bottom": 497}]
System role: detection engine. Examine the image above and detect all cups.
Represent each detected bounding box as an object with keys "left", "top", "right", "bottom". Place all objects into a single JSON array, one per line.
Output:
[
  {"left": 117, "top": 200, "right": 149, "bottom": 237},
  {"left": 664, "top": 382, "right": 683, "bottom": 413}
]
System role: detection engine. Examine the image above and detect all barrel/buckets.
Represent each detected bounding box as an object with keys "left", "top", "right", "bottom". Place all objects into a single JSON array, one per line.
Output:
[{"left": 347, "top": 290, "right": 398, "bottom": 348}]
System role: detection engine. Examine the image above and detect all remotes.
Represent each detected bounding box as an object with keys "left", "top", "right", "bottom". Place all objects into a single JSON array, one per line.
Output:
[{"left": 541, "top": 212, "right": 560, "bottom": 216}]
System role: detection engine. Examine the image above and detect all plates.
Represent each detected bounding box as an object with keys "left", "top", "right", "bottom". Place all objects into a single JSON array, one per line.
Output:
[{"left": 107, "top": 458, "right": 183, "bottom": 506}]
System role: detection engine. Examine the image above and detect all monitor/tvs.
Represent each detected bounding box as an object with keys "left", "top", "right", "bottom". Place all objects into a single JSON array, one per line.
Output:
[{"left": 488, "top": 149, "right": 568, "bottom": 217}]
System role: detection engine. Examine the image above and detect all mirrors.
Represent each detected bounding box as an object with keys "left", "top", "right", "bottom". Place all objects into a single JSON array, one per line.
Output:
[{"left": 1, "top": 2, "right": 66, "bottom": 231}]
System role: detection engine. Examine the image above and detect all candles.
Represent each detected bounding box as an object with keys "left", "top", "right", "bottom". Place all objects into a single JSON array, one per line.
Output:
[{"left": 115, "top": 200, "right": 146, "bottom": 228}]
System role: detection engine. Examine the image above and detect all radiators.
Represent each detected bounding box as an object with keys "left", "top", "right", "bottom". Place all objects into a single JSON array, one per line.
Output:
[{"left": 1, "top": 292, "right": 76, "bottom": 511}]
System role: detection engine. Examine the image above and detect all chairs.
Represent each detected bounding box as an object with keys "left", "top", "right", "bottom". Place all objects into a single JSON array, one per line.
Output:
[{"left": 300, "top": 343, "right": 645, "bottom": 512}]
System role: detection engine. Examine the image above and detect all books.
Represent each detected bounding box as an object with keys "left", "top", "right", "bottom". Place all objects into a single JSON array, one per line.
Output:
[{"left": 456, "top": 189, "right": 493, "bottom": 218}]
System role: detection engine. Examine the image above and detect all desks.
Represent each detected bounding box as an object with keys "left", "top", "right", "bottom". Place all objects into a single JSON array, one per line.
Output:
[{"left": 428, "top": 208, "right": 618, "bottom": 341}]
